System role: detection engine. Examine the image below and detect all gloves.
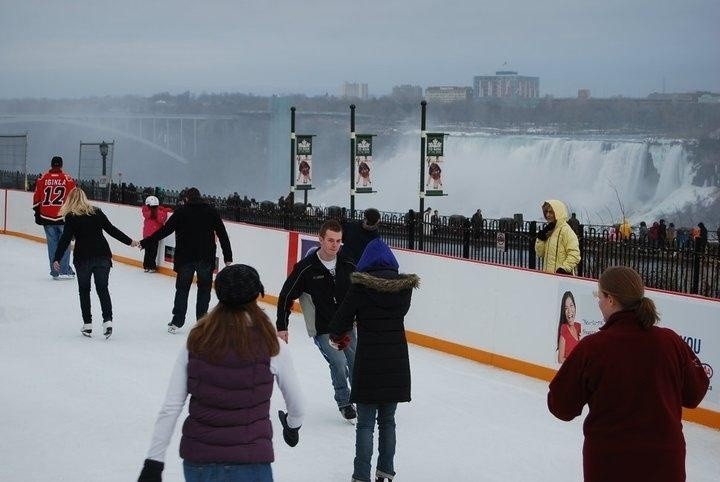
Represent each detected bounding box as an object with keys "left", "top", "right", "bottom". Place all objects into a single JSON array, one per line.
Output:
[
  {"left": 537, "top": 223, "right": 553, "bottom": 240},
  {"left": 557, "top": 268, "right": 568, "bottom": 274},
  {"left": 328, "top": 332, "right": 351, "bottom": 352},
  {"left": 35, "top": 212, "right": 39, "bottom": 224},
  {"left": 279, "top": 412, "right": 301, "bottom": 448},
  {"left": 139, "top": 460, "right": 163, "bottom": 481}
]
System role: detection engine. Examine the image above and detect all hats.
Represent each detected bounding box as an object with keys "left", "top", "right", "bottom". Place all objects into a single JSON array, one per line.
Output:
[
  {"left": 51, "top": 156, "right": 63, "bottom": 166},
  {"left": 214, "top": 264, "right": 264, "bottom": 306},
  {"left": 146, "top": 196, "right": 159, "bottom": 205},
  {"left": 364, "top": 209, "right": 380, "bottom": 219}
]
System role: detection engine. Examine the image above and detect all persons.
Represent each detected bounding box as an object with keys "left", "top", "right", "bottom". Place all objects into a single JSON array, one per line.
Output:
[
  {"left": 555, "top": 290, "right": 588, "bottom": 365},
  {"left": 225, "top": 192, "right": 262, "bottom": 222},
  {"left": 432, "top": 210, "right": 442, "bottom": 242},
  {"left": 278, "top": 196, "right": 285, "bottom": 222},
  {"left": 285, "top": 192, "right": 294, "bottom": 222},
  {"left": 535, "top": 200, "right": 581, "bottom": 277},
  {"left": 567, "top": 213, "right": 581, "bottom": 250},
  {"left": 340, "top": 208, "right": 381, "bottom": 266},
  {"left": 142, "top": 196, "right": 174, "bottom": 273},
  {"left": 546, "top": 266, "right": 710, "bottom": 482},
  {"left": 638, "top": 222, "right": 650, "bottom": 250},
  {"left": 471, "top": 209, "right": 483, "bottom": 251},
  {"left": 666, "top": 223, "right": 677, "bottom": 257},
  {"left": 1, "top": 170, "right": 42, "bottom": 192},
  {"left": 137, "top": 188, "right": 234, "bottom": 333},
  {"left": 650, "top": 222, "right": 660, "bottom": 251},
  {"left": 275, "top": 220, "right": 360, "bottom": 426},
  {"left": 304, "top": 203, "right": 314, "bottom": 225},
  {"left": 695, "top": 223, "right": 708, "bottom": 265},
  {"left": 137, "top": 264, "right": 304, "bottom": 482},
  {"left": 33, "top": 156, "right": 78, "bottom": 280},
  {"left": 328, "top": 240, "right": 421, "bottom": 482},
  {"left": 617, "top": 218, "right": 632, "bottom": 253},
  {"left": 424, "top": 207, "right": 432, "bottom": 242},
  {"left": 608, "top": 225, "right": 617, "bottom": 242},
  {"left": 53, "top": 188, "right": 137, "bottom": 339},
  {"left": 659, "top": 219, "right": 668, "bottom": 253},
  {"left": 69, "top": 177, "right": 188, "bottom": 207}
]
